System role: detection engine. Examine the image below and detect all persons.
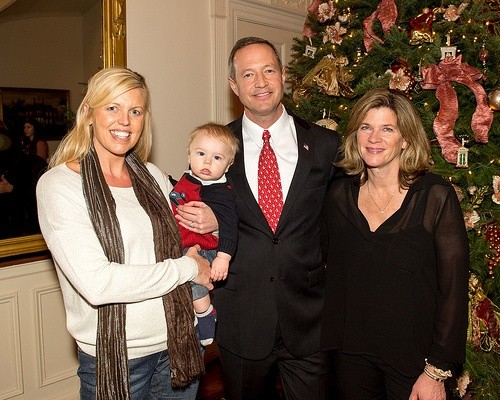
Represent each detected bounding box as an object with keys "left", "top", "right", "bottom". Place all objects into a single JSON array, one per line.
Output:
[
  {"left": 36, "top": 67, "right": 221, "bottom": 400},
  {"left": 164, "top": 123, "right": 240, "bottom": 347},
  {"left": 22, "top": 118, "right": 50, "bottom": 160},
  {"left": 211, "top": 37, "right": 347, "bottom": 400},
  {"left": 0, "top": 148, "right": 29, "bottom": 238},
  {"left": 322, "top": 87, "right": 469, "bottom": 400}
]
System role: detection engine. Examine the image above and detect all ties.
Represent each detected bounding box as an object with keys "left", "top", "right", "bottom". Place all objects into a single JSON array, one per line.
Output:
[{"left": 258, "top": 130, "right": 284, "bottom": 235}]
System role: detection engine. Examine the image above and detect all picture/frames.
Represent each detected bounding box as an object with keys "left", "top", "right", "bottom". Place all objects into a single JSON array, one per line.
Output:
[{"left": 0, "top": 0, "right": 126, "bottom": 259}]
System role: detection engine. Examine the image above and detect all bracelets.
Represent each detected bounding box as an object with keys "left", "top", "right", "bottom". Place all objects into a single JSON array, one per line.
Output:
[{"left": 424, "top": 364, "right": 452, "bottom": 382}]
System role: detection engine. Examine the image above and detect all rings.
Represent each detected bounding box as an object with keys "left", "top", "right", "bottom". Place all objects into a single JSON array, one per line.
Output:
[{"left": 192, "top": 221, "right": 194, "bottom": 228}]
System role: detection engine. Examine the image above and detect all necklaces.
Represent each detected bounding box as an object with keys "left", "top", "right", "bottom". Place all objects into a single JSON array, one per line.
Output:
[{"left": 367, "top": 182, "right": 394, "bottom": 214}]
